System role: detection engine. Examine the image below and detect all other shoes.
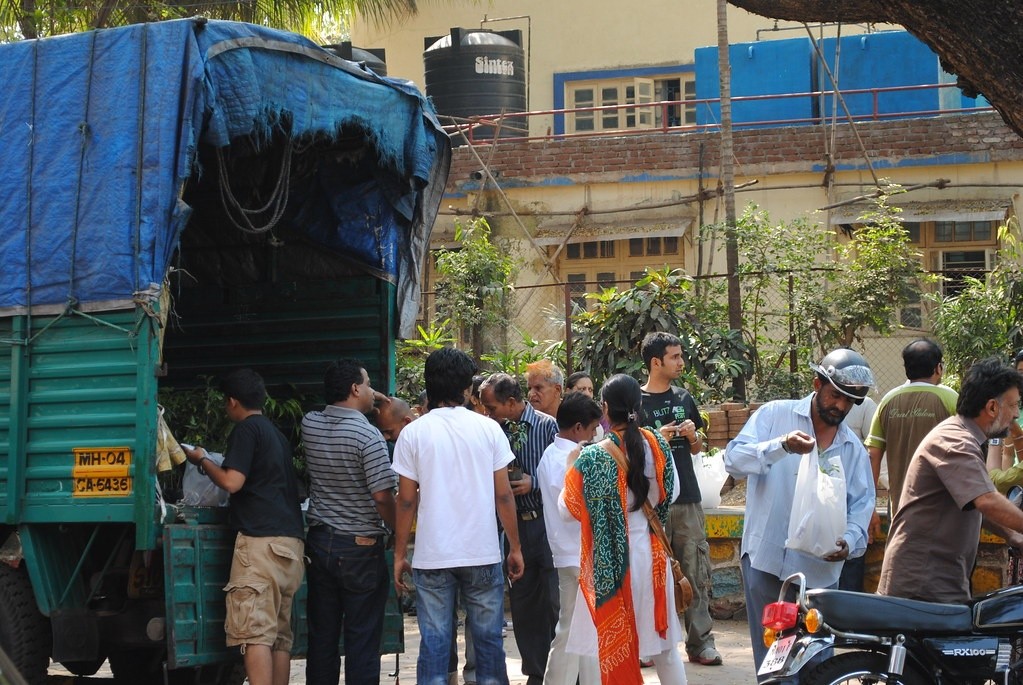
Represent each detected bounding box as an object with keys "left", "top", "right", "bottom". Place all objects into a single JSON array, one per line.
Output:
[
  {"left": 639, "top": 658, "right": 654, "bottom": 668},
  {"left": 501, "top": 619, "right": 514, "bottom": 630},
  {"left": 690, "top": 648, "right": 723, "bottom": 666},
  {"left": 501, "top": 629, "right": 507, "bottom": 637},
  {"left": 408, "top": 601, "right": 417, "bottom": 616}
]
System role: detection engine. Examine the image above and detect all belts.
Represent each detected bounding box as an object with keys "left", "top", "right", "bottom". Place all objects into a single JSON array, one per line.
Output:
[
  {"left": 516, "top": 508, "right": 543, "bottom": 521},
  {"left": 310, "top": 524, "right": 353, "bottom": 536}
]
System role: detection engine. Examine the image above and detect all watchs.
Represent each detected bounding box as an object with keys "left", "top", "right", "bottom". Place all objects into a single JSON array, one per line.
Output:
[{"left": 989, "top": 438, "right": 1002, "bottom": 447}]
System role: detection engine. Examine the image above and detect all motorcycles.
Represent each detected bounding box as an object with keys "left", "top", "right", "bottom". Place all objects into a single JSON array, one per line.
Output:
[{"left": 756, "top": 485, "right": 1022, "bottom": 685}]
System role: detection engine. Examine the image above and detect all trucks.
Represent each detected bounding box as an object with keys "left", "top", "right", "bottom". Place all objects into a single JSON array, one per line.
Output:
[{"left": 0, "top": 17, "right": 454, "bottom": 684}]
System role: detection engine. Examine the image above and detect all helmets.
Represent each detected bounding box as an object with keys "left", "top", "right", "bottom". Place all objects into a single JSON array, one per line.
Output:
[{"left": 810, "top": 348, "right": 869, "bottom": 405}]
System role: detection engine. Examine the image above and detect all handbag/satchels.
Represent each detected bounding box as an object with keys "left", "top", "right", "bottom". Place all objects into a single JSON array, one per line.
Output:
[
  {"left": 670, "top": 557, "right": 693, "bottom": 615},
  {"left": 690, "top": 447, "right": 730, "bottom": 510},
  {"left": 181, "top": 453, "right": 231, "bottom": 506},
  {"left": 784, "top": 438, "right": 847, "bottom": 559}
]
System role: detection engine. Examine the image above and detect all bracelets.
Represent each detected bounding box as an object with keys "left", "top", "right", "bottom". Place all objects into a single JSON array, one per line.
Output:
[
  {"left": 1015, "top": 448, "right": 1023, "bottom": 452},
  {"left": 1012, "top": 435, "right": 1023, "bottom": 440},
  {"left": 688, "top": 432, "right": 698, "bottom": 445},
  {"left": 197, "top": 456, "right": 213, "bottom": 475},
  {"left": 1004, "top": 443, "right": 1014, "bottom": 448}
]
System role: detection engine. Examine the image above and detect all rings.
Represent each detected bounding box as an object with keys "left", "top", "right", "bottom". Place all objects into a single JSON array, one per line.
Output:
[{"left": 669, "top": 432, "right": 673, "bottom": 438}]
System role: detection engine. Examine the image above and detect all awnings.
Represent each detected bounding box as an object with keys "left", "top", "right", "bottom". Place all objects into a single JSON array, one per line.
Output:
[{"left": 427, "top": 199, "right": 1012, "bottom": 252}]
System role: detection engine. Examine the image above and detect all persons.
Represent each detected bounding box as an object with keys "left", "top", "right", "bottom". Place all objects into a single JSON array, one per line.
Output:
[
  {"left": 986, "top": 350, "right": 1023, "bottom": 493},
  {"left": 523, "top": 360, "right": 562, "bottom": 418},
  {"left": 465, "top": 370, "right": 515, "bottom": 638},
  {"left": 862, "top": 339, "right": 960, "bottom": 543},
  {"left": 418, "top": 387, "right": 432, "bottom": 418},
  {"left": 724, "top": 349, "right": 875, "bottom": 685},
  {"left": 391, "top": 347, "right": 525, "bottom": 685},
  {"left": 480, "top": 373, "right": 560, "bottom": 685},
  {"left": 636, "top": 332, "right": 724, "bottom": 668},
  {"left": 183, "top": 369, "right": 305, "bottom": 685},
  {"left": 536, "top": 392, "right": 602, "bottom": 685},
  {"left": 557, "top": 374, "right": 687, "bottom": 685},
  {"left": 380, "top": 395, "right": 422, "bottom": 446},
  {"left": 875, "top": 356, "right": 1023, "bottom": 603},
  {"left": 564, "top": 371, "right": 604, "bottom": 443},
  {"left": 300, "top": 365, "right": 397, "bottom": 685},
  {"left": 822, "top": 396, "right": 881, "bottom": 454}
]
